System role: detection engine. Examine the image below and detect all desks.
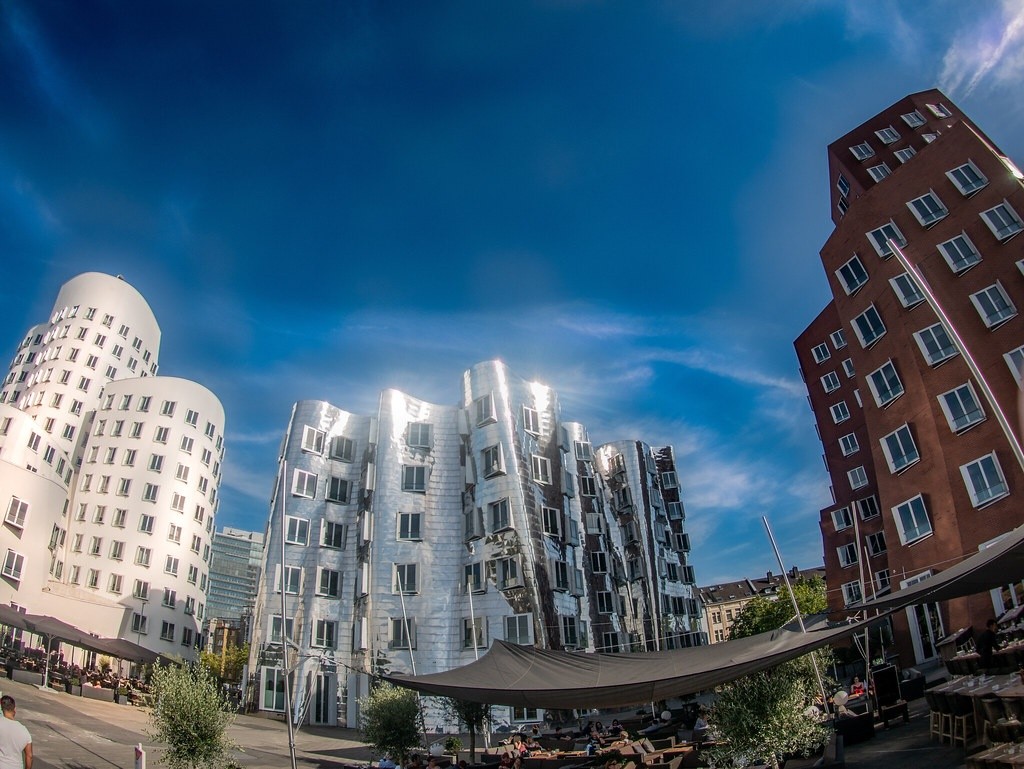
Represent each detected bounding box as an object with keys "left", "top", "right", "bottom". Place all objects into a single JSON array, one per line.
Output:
[
  {"left": 943, "top": 638, "right": 1024, "bottom": 676},
  {"left": 922, "top": 673, "right": 1024, "bottom": 714},
  {"left": 998, "top": 604, "right": 1024, "bottom": 627},
  {"left": 963, "top": 743, "right": 1024, "bottom": 769},
  {"left": 936, "top": 624, "right": 972, "bottom": 667},
  {"left": 621, "top": 714, "right": 652, "bottom": 727}
]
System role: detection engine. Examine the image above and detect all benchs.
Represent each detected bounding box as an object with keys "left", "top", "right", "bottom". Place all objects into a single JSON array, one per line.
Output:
[
  {"left": 81, "top": 685, "right": 114, "bottom": 702},
  {"left": 900, "top": 667, "right": 947, "bottom": 701},
  {"left": 12, "top": 668, "right": 43, "bottom": 686},
  {"left": 824, "top": 702, "right": 876, "bottom": 745}
]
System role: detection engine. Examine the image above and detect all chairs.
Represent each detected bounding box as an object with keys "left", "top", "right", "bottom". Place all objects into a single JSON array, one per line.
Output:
[
  {"left": 959, "top": 637, "right": 975, "bottom": 652},
  {"left": 928, "top": 697, "right": 1024, "bottom": 747}
]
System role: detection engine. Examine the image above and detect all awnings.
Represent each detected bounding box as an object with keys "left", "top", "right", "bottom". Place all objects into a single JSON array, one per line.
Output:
[
  {"left": 371, "top": 593, "right": 929, "bottom": 707},
  {"left": 849, "top": 523, "right": 1024, "bottom": 609}
]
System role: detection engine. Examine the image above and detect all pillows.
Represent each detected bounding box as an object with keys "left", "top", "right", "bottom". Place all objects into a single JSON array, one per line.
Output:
[
  {"left": 632, "top": 743, "right": 647, "bottom": 755},
  {"left": 643, "top": 737, "right": 655, "bottom": 753},
  {"left": 513, "top": 750, "right": 520, "bottom": 757},
  {"left": 496, "top": 747, "right": 506, "bottom": 755},
  {"left": 541, "top": 734, "right": 553, "bottom": 739},
  {"left": 563, "top": 731, "right": 573, "bottom": 737}
]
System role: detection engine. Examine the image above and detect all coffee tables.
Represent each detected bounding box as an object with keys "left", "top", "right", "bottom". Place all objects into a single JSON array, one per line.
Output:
[
  {"left": 847, "top": 692, "right": 873, "bottom": 710},
  {"left": 601, "top": 734, "right": 620, "bottom": 746},
  {"left": 556, "top": 751, "right": 587, "bottom": 759},
  {"left": 663, "top": 742, "right": 693, "bottom": 763},
  {"left": 537, "top": 750, "right": 564, "bottom": 758}
]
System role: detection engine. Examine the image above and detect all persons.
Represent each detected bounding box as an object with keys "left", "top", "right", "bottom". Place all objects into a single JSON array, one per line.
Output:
[
  {"left": 0, "top": 695, "right": 32, "bottom": 769},
  {"left": 0, "top": 631, "right": 154, "bottom": 694},
  {"left": 849, "top": 668, "right": 874, "bottom": 693},
  {"left": 975, "top": 618, "right": 1008, "bottom": 676},
  {"left": 378, "top": 751, "right": 525, "bottom": 769},
  {"left": 503, "top": 701, "right": 718, "bottom": 756}
]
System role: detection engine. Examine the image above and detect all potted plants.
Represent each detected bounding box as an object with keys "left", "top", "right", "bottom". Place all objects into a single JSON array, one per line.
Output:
[
  {"left": 68, "top": 677, "right": 81, "bottom": 696},
  {"left": 116, "top": 687, "right": 128, "bottom": 705}
]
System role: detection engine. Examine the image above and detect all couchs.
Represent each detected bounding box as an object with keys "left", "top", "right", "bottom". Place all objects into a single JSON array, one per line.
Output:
[
  {"left": 419, "top": 718, "right": 685, "bottom": 769},
  {"left": 783, "top": 735, "right": 845, "bottom": 769}
]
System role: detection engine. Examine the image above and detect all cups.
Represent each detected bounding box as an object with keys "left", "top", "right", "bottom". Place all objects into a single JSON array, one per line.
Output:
[
  {"left": 956, "top": 649, "right": 965, "bottom": 659},
  {"left": 992, "top": 685, "right": 999, "bottom": 691}
]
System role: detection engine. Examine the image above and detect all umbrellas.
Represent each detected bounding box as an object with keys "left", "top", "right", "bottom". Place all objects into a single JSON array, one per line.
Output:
[
  {"left": 0, "top": 604, "right": 27, "bottom": 630},
  {"left": 20, "top": 614, "right": 185, "bottom": 704}
]
